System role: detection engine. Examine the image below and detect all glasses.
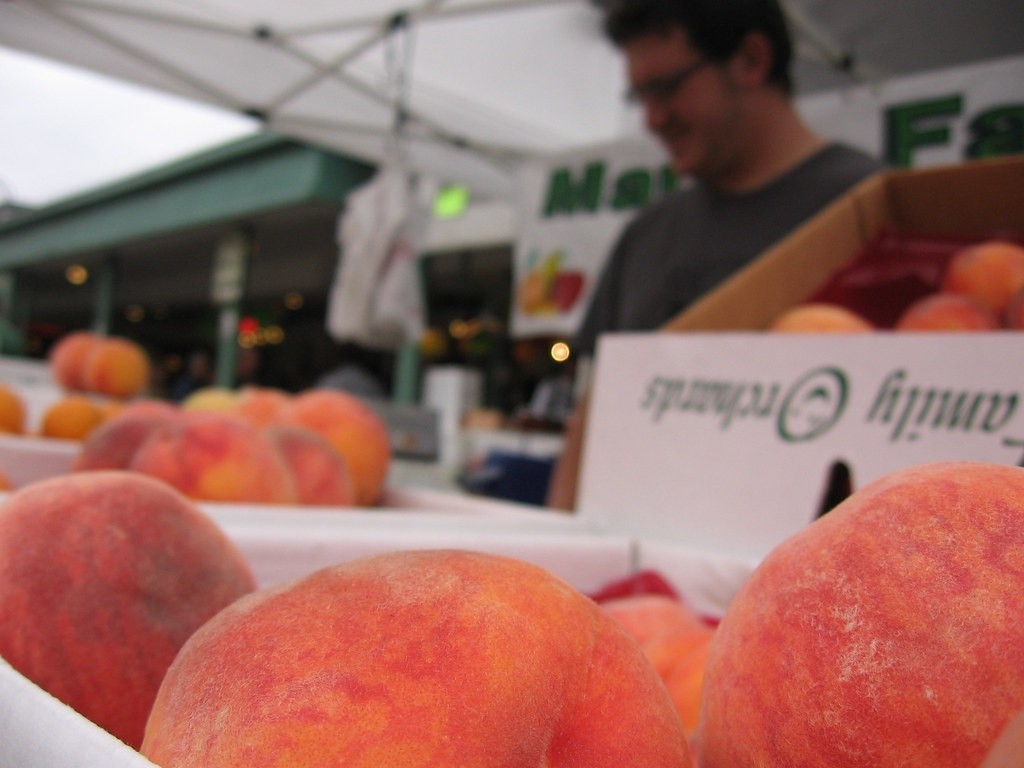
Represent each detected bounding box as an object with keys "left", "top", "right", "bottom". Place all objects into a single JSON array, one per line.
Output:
[{"left": 626, "top": 59, "right": 709, "bottom": 105}]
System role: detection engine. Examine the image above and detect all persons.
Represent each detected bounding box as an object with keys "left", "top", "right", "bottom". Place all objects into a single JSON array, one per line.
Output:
[{"left": 548, "top": 0, "right": 894, "bottom": 359}]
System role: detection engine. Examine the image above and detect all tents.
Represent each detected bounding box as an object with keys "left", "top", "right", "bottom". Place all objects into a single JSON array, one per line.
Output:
[{"left": 0, "top": 0, "right": 1024, "bottom": 186}]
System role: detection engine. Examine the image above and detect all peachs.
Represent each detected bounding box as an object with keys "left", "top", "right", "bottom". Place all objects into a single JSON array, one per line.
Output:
[{"left": 0, "top": 242, "right": 1024, "bottom": 768}]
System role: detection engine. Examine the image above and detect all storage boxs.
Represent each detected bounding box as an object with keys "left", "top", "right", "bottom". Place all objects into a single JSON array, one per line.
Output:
[{"left": 649, "top": 154, "right": 1024, "bottom": 335}]
